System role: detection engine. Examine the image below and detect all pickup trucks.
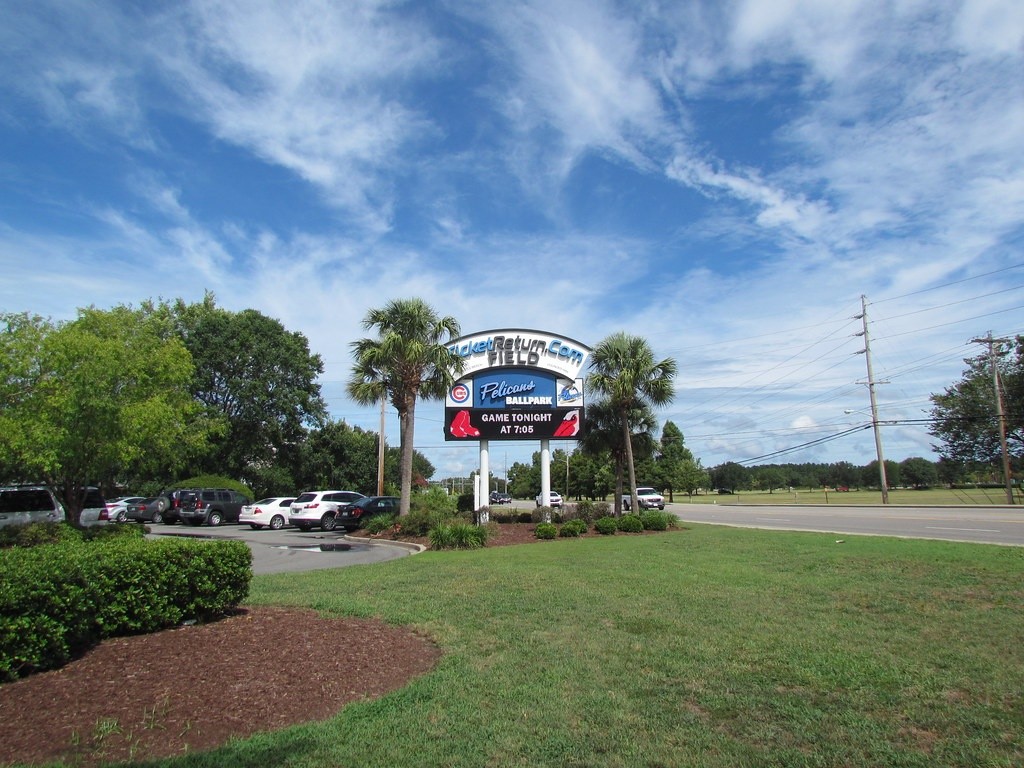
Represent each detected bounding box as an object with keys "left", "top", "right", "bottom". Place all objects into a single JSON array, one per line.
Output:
[{"left": 623, "top": 487, "right": 666, "bottom": 512}]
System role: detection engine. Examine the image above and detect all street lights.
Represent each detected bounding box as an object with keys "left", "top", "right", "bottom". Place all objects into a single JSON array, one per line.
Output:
[{"left": 844, "top": 408, "right": 891, "bottom": 509}]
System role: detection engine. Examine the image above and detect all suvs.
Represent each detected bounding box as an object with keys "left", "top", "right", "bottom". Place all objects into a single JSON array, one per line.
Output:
[
  {"left": 155, "top": 487, "right": 203, "bottom": 527},
  {"left": 174, "top": 487, "right": 252, "bottom": 526},
  {"left": 288, "top": 490, "right": 367, "bottom": 533}
]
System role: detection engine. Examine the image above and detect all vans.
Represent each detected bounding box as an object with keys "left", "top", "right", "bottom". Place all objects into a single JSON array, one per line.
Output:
[{"left": 0, "top": 483, "right": 112, "bottom": 532}]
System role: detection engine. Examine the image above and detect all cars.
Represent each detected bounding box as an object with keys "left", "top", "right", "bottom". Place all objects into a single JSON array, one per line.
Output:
[
  {"left": 333, "top": 496, "right": 414, "bottom": 532},
  {"left": 535, "top": 491, "right": 563, "bottom": 510},
  {"left": 126, "top": 497, "right": 171, "bottom": 524},
  {"left": 238, "top": 496, "right": 297, "bottom": 530},
  {"left": 489, "top": 491, "right": 512, "bottom": 505},
  {"left": 104, "top": 496, "right": 146, "bottom": 523}
]
